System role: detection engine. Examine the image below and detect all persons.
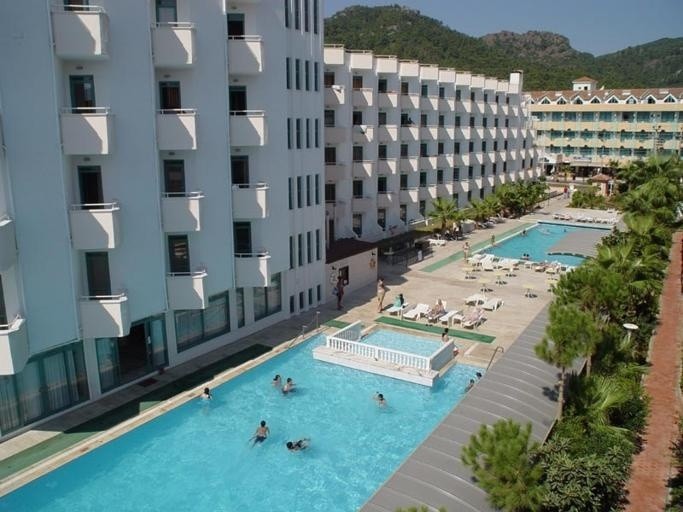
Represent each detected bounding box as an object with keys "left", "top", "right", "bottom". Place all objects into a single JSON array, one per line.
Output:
[
  {"left": 270, "top": 374, "right": 282, "bottom": 391},
  {"left": 200, "top": 388, "right": 212, "bottom": 401},
  {"left": 372, "top": 392, "right": 385, "bottom": 406},
  {"left": 334, "top": 274, "right": 344, "bottom": 309},
  {"left": 427, "top": 298, "right": 444, "bottom": 318},
  {"left": 461, "top": 242, "right": 470, "bottom": 261},
  {"left": 464, "top": 379, "right": 474, "bottom": 392},
  {"left": 375, "top": 275, "right": 385, "bottom": 314},
  {"left": 475, "top": 372, "right": 482, "bottom": 380},
  {"left": 441, "top": 327, "right": 449, "bottom": 342},
  {"left": 280, "top": 377, "right": 296, "bottom": 395},
  {"left": 285, "top": 437, "right": 310, "bottom": 452},
  {"left": 248, "top": 421, "right": 270, "bottom": 448}
]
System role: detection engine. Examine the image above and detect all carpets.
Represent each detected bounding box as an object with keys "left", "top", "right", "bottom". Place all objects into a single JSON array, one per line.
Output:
[
  {"left": 418, "top": 222, "right": 536, "bottom": 272},
  {"left": 137, "top": 377, "right": 158, "bottom": 387},
  {"left": 321, "top": 318, "right": 351, "bottom": 329},
  {"left": 0, "top": 342, "right": 272, "bottom": 483},
  {"left": 375, "top": 316, "right": 496, "bottom": 344}
]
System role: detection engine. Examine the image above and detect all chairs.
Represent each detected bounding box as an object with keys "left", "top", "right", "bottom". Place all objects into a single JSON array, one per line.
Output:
[
  {"left": 387, "top": 253, "right": 577, "bottom": 329},
  {"left": 552, "top": 207, "right": 622, "bottom": 225}
]
